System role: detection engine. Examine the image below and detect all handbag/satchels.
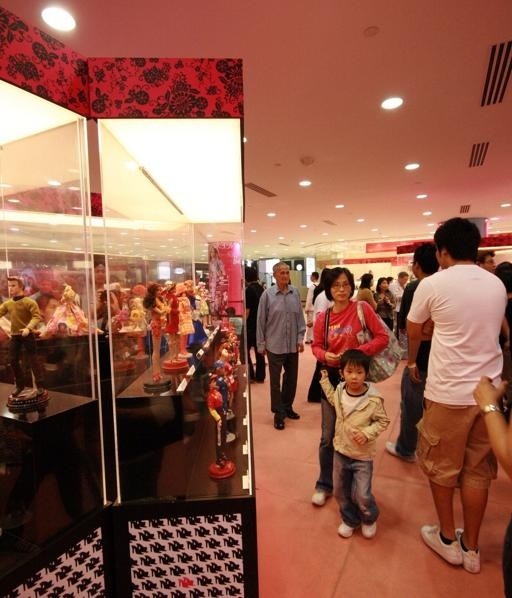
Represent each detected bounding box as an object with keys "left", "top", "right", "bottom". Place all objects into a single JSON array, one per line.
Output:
[
  {"left": 308, "top": 360, "right": 322, "bottom": 403},
  {"left": 356, "top": 312, "right": 404, "bottom": 383}
]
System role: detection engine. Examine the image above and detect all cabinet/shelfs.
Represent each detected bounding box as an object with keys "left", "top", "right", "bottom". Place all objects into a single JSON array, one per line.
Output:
[{"left": 1, "top": 7, "right": 261, "bottom": 598}]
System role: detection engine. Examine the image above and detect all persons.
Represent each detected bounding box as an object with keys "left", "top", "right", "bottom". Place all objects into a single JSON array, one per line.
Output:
[
  {"left": 318, "top": 348, "right": 392, "bottom": 539},
  {"left": 1, "top": 275, "right": 47, "bottom": 396},
  {"left": 206, "top": 244, "right": 227, "bottom": 313},
  {"left": 406, "top": 217, "right": 511, "bottom": 575},
  {"left": 47, "top": 283, "right": 105, "bottom": 337},
  {"left": 107, "top": 279, "right": 242, "bottom": 480},
  {"left": 385, "top": 243, "right": 511, "bottom": 480},
  {"left": 243, "top": 261, "right": 407, "bottom": 431},
  {"left": 471, "top": 376, "right": 512, "bottom": 597}
]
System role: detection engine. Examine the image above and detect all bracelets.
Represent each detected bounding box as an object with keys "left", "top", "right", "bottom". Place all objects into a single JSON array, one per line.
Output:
[{"left": 478, "top": 404, "right": 504, "bottom": 416}]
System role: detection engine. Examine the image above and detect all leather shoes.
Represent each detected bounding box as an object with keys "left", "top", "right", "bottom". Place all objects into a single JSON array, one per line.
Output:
[
  {"left": 285, "top": 410, "right": 300, "bottom": 419},
  {"left": 274, "top": 416, "right": 284, "bottom": 429}
]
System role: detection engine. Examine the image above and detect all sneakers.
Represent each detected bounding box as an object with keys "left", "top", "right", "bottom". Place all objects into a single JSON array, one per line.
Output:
[
  {"left": 338, "top": 521, "right": 361, "bottom": 537},
  {"left": 312, "top": 488, "right": 333, "bottom": 506},
  {"left": 421, "top": 525, "right": 463, "bottom": 565},
  {"left": 361, "top": 521, "right": 377, "bottom": 538},
  {"left": 386, "top": 441, "right": 417, "bottom": 462},
  {"left": 455, "top": 528, "right": 480, "bottom": 573},
  {"left": 249, "top": 379, "right": 264, "bottom": 383}
]
